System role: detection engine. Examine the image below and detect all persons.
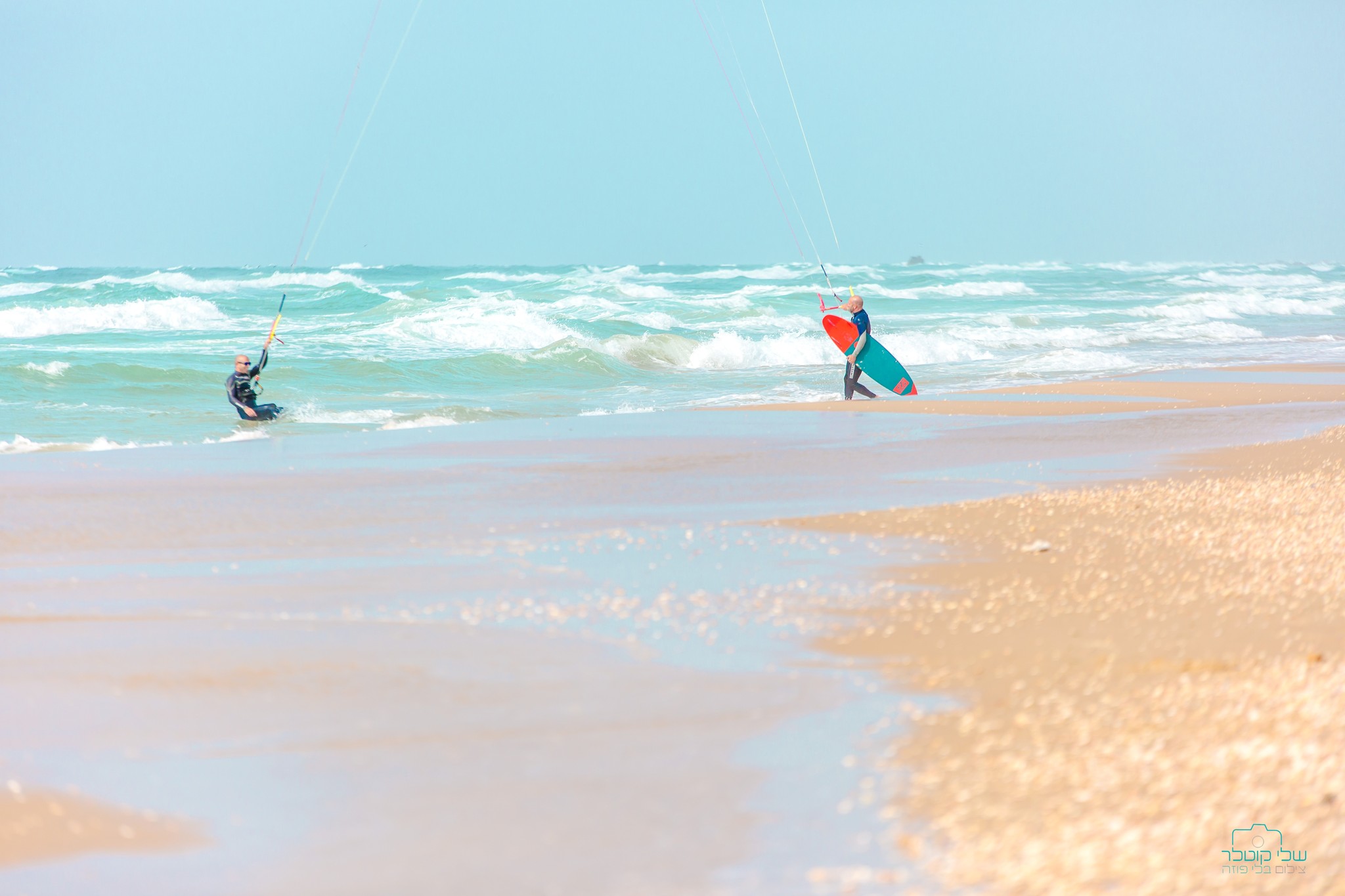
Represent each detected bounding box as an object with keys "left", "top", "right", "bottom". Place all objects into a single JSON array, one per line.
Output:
[
  {"left": 840, "top": 296, "right": 881, "bottom": 400},
  {"left": 226, "top": 340, "right": 283, "bottom": 421}
]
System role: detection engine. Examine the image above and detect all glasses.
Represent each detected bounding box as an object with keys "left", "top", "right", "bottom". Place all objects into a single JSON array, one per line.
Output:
[{"left": 239, "top": 362, "right": 250, "bottom": 365}]
